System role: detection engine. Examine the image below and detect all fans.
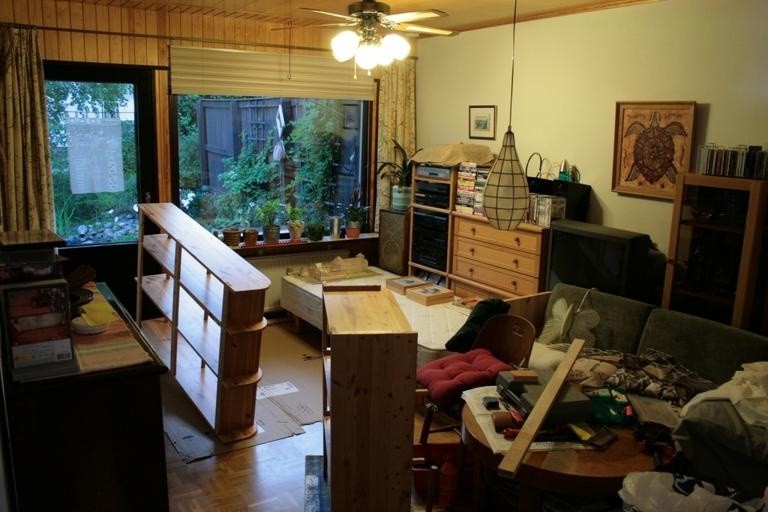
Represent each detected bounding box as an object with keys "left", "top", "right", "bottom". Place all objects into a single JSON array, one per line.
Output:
[{"left": 271, "top": 0, "right": 452, "bottom": 37}]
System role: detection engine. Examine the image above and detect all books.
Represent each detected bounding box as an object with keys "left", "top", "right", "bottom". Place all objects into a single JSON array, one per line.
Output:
[
  {"left": 454, "top": 162, "right": 494, "bottom": 217},
  {"left": 700, "top": 145, "right": 763, "bottom": 179}
]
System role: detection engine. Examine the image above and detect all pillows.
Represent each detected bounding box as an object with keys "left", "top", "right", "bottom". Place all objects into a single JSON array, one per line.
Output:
[{"left": 416, "top": 349, "right": 513, "bottom": 401}]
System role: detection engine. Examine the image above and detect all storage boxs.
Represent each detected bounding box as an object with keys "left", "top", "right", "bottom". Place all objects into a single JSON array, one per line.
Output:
[{"left": 410, "top": 441, "right": 503, "bottom": 492}]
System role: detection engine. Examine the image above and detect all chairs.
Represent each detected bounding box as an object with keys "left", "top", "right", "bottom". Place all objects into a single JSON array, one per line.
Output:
[{"left": 421, "top": 315, "right": 536, "bottom": 445}]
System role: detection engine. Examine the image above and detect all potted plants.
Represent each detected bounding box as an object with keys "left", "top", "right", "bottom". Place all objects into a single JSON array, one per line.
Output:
[
  {"left": 223, "top": 199, "right": 370, "bottom": 246},
  {"left": 381, "top": 139, "right": 422, "bottom": 211}
]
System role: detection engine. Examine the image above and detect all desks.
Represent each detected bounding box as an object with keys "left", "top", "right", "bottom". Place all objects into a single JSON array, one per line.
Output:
[
  {"left": 280, "top": 257, "right": 472, "bottom": 369},
  {"left": 461, "top": 382, "right": 681, "bottom": 512}
]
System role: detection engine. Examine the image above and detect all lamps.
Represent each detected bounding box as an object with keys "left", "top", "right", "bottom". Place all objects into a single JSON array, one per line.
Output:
[
  {"left": 482, "top": 1, "right": 530, "bottom": 231},
  {"left": 330, "top": 26, "right": 411, "bottom": 79}
]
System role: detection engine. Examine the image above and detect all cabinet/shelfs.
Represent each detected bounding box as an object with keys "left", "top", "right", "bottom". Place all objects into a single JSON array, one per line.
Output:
[
  {"left": 377, "top": 209, "right": 407, "bottom": 276},
  {"left": 452, "top": 211, "right": 549, "bottom": 301},
  {"left": 1, "top": 278, "right": 168, "bottom": 512},
  {"left": 409, "top": 159, "right": 450, "bottom": 292},
  {"left": 661, "top": 172, "right": 768, "bottom": 330},
  {"left": 320, "top": 285, "right": 418, "bottom": 512},
  {"left": 133, "top": 200, "right": 272, "bottom": 446}
]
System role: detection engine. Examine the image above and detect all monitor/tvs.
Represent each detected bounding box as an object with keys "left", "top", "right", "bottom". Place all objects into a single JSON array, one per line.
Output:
[{"left": 544, "top": 220, "right": 667, "bottom": 306}]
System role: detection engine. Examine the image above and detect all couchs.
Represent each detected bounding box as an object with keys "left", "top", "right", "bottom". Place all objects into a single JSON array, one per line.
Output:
[{"left": 501, "top": 283, "right": 768, "bottom": 512}]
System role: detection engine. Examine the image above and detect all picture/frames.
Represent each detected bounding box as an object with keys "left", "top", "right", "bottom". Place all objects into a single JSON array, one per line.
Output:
[
  {"left": 468, "top": 105, "right": 496, "bottom": 139},
  {"left": 611, "top": 101, "right": 696, "bottom": 200}
]
